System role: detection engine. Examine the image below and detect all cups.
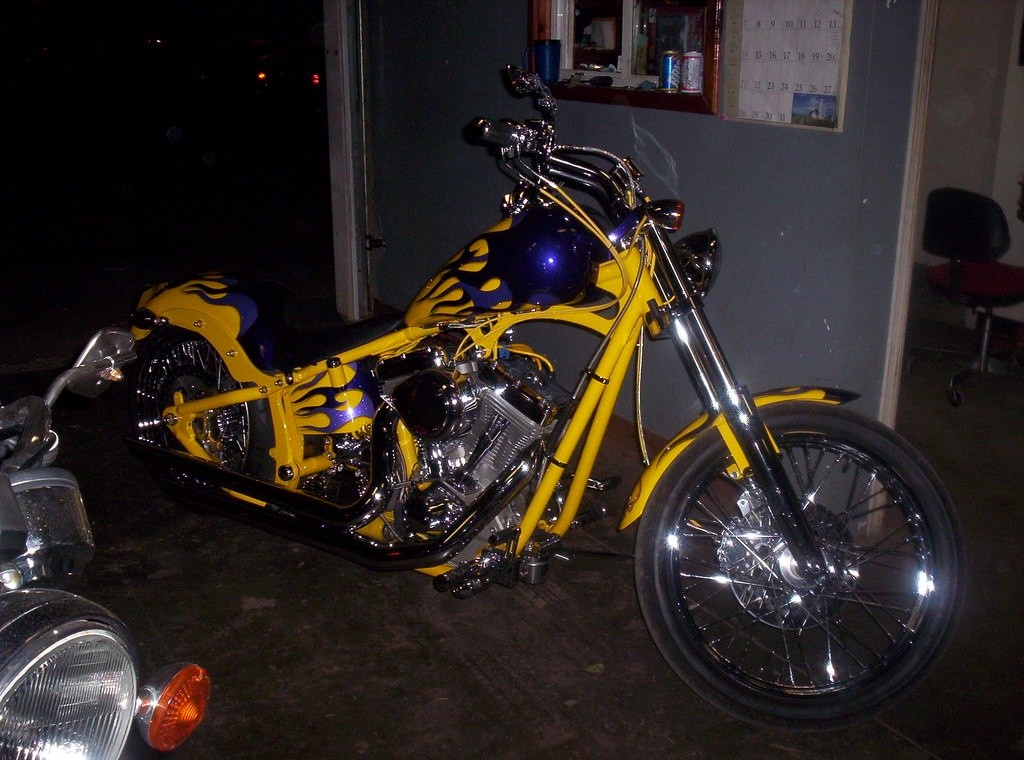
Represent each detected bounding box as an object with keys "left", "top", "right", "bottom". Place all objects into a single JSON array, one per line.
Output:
[{"left": 522, "top": 38, "right": 562, "bottom": 85}]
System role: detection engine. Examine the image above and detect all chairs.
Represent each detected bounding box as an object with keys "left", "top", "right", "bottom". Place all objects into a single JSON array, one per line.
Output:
[{"left": 920, "top": 187, "right": 1024, "bottom": 406}]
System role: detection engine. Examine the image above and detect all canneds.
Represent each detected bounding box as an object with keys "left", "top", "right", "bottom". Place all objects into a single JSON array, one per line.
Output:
[
  {"left": 657, "top": 50, "right": 681, "bottom": 93},
  {"left": 680, "top": 51, "right": 703, "bottom": 93}
]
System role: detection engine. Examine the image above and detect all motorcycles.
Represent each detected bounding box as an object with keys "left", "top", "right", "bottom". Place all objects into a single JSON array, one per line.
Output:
[
  {"left": 0, "top": 326, "right": 212, "bottom": 760},
  {"left": 43, "top": 62, "right": 967, "bottom": 734}
]
